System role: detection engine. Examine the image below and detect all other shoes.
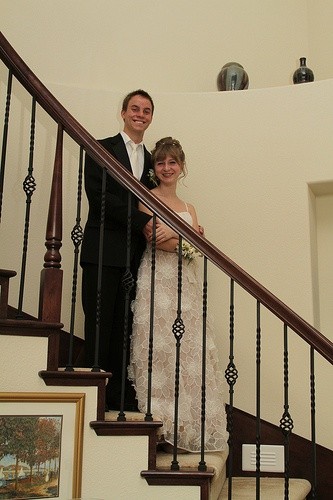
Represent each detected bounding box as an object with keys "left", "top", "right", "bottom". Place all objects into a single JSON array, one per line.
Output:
[{"left": 164, "top": 442, "right": 187, "bottom": 454}]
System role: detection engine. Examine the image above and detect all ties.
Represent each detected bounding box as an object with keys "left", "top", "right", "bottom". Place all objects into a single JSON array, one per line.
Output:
[{"left": 131, "top": 144, "right": 140, "bottom": 178}]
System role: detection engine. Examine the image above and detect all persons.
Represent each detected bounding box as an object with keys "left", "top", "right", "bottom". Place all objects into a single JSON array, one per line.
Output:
[
  {"left": 80, "top": 90, "right": 204, "bottom": 411},
  {"left": 126, "top": 136, "right": 229, "bottom": 454}
]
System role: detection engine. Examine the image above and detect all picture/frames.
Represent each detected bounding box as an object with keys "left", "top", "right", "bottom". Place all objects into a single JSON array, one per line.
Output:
[{"left": 0, "top": 391, "right": 86, "bottom": 500}]
